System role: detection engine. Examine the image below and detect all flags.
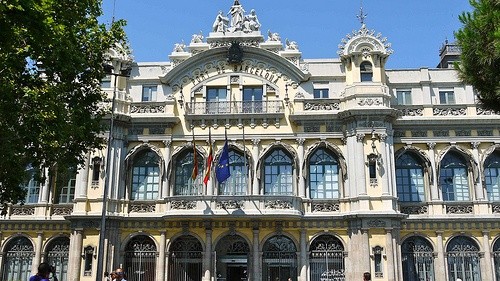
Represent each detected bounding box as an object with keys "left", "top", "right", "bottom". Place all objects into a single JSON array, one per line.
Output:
[
  {"left": 191, "top": 129, "right": 198, "bottom": 181},
  {"left": 215, "top": 128, "right": 231, "bottom": 184},
  {"left": 203, "top": 128, "right": 213, "bottom": 185}
]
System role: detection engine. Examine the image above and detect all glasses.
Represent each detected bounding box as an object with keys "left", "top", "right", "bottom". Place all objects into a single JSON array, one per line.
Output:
[{"left": 116, "top": 272, "right": 124, "bottom": 275}]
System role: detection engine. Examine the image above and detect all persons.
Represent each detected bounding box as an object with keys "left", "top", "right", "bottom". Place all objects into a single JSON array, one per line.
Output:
[
  {"left": 103, "top": 268, "right": 128, "bottom": 281},
  {"left": 363, "top": 272, "right": 372, "bottom": 281},
  {"left": 213, "top": 0, "right": 261, "bottom": 33},
  {"left": 29, "top": 262, "right": 58, "bottom": 281}
]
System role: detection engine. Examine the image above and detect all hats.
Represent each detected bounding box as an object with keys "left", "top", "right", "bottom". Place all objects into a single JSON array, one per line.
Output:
[{"left": 110, "top": 271, "right": 116, "bottom": 275}]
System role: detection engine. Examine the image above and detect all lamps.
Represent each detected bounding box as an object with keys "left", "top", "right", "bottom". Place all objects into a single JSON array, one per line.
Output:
[{"left": 370, "top": 129, "right": 377, "bottom": 149}]
